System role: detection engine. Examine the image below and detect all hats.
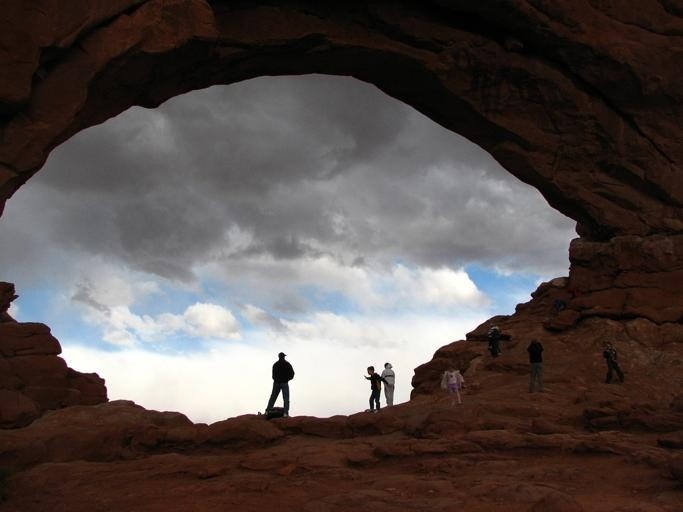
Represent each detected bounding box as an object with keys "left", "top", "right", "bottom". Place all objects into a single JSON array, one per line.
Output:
[{"left": 278, "top": 352, "right": 286, "bottom": 358}]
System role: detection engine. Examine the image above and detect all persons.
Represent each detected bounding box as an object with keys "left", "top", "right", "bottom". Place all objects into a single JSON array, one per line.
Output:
[
  {"left": 380, "top": 363, "right": 395, "bottom": 407},
  {"left": 487, "top": 323, "right": 501, "bottom": 358},
  {"left": 440, "top": 364, "right": 465, "bottom": 404},
  {"left": 526, "top": 338, "right": 545, "bottom": 393},
  {"left": 363, "top": 366, "right": 391, "bottom": 413},
  {"left": 264, "top": 351, "right": 295, "bottom": 417},
  {"left": 600, "top": 342, "right": 625, "bottom": 385}
]
